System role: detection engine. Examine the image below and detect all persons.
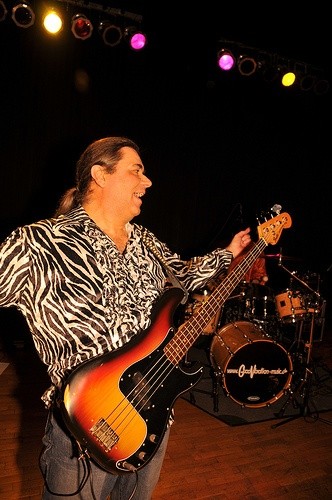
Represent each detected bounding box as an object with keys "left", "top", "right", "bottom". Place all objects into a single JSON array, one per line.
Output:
[{"left": 0, "top": 136, "right": 251, "bottom": 500}]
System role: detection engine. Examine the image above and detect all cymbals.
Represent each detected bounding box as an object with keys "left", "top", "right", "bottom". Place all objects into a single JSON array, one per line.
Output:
[{"left": 260, "top": 254, "right": 290, "bottom": 258}]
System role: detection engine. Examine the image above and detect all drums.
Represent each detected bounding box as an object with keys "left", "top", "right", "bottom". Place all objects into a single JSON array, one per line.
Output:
[
  {"left": 228, "top": 252, "right": 268, "bottom": 286},
  {"left": 209, "top": 321, "right": 294, "bottom": 408},
  {"left": 274, "top": 287, "right": 320, "bottom": 323},
  {"left": 185, "top": 294, "right": 221, "bottom": 335},
  {"left": 249, "top": 296, "right": 276, "bottom": 321}
]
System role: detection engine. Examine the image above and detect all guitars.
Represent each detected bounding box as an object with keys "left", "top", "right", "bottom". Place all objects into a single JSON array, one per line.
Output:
[{"left": 52, "top": 204, "right": 292, "bottom": 477}]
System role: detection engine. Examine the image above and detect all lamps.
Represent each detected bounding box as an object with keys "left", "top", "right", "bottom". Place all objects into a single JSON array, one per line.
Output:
[
  {"left": 215, "top": 47, "right": 329, "bottom": 96},
  {"left": 0, "top": 0, "right": 147, "bottom": 52}
]
